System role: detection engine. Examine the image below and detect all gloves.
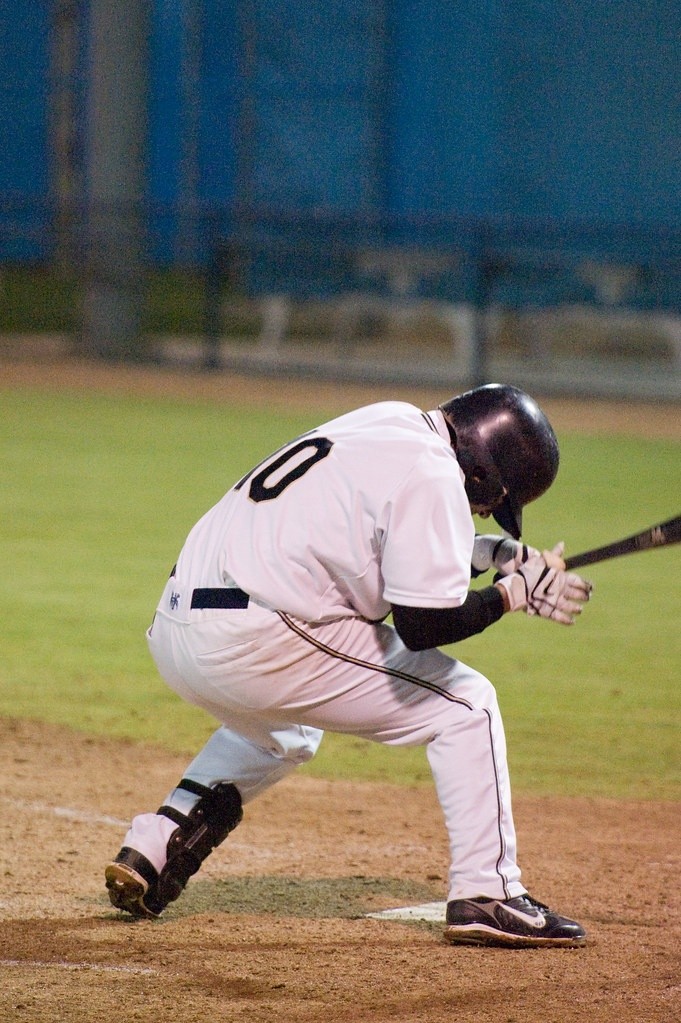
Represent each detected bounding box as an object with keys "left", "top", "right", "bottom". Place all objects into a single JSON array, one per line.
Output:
[{"left": 496, "top": 542, "right": 594, "bottom": 627}]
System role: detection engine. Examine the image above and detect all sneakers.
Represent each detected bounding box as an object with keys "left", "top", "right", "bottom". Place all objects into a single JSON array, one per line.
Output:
[
  {"left": 103, "top": 846, "right": 161, "bottom": 920},
  {"left": 442, "top": 892, "right": 587, "bottom": 945}
]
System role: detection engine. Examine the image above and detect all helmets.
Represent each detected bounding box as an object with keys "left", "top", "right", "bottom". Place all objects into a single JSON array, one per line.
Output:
[{"left": 437, "top": 382, "right": 561, "bottom": 541}]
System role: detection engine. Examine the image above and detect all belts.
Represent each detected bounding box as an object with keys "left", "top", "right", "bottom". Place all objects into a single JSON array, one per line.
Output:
[{"left": 169, "top": 563, "right": 249, "bottom": 609}]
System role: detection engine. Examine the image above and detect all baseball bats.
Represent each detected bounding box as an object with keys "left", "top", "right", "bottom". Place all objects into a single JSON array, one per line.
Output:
[{"left": 493, "top": 513, "right": 681, "bottom": 584}]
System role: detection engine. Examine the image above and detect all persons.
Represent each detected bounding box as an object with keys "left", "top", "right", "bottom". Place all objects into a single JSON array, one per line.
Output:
[{"left": 104, "top": 383, "right": 593, "bottom": 949}]
